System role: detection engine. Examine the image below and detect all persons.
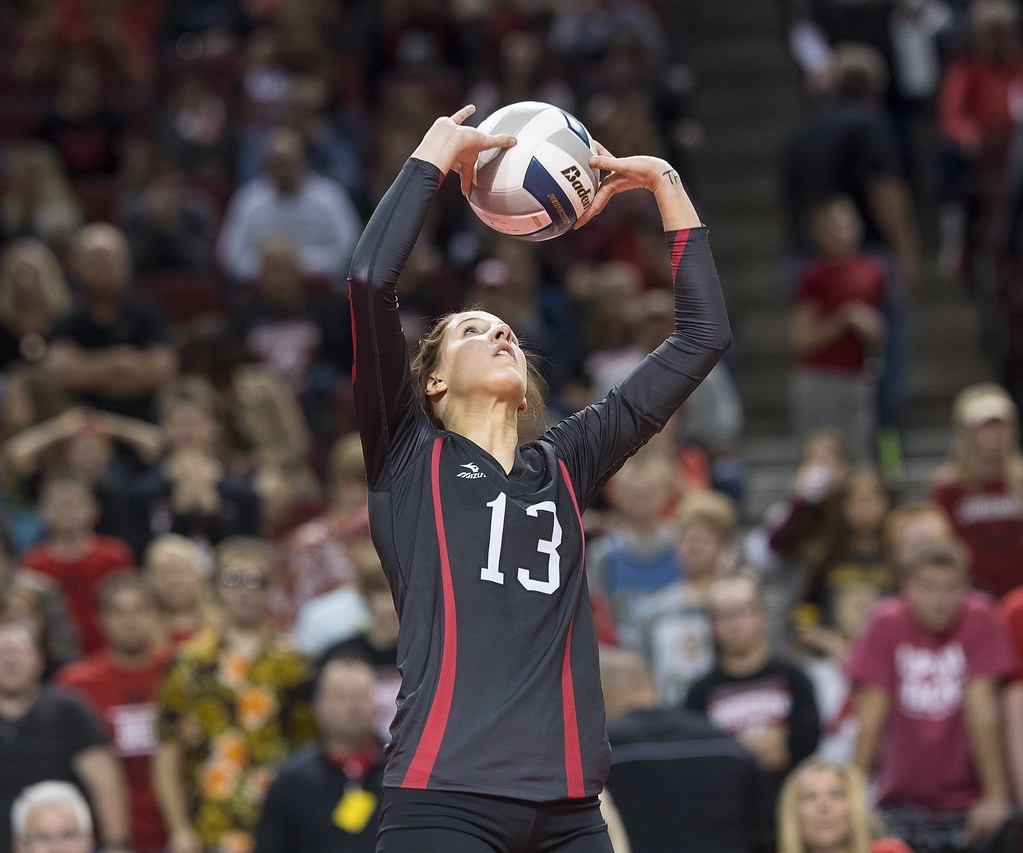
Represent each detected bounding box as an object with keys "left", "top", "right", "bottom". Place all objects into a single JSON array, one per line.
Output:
[
  {"left": 1, "top": 0, "right": 1023, "bottom": 853},
  {"left": 347, "top": 102, "right": 734, "bottom": 852}
]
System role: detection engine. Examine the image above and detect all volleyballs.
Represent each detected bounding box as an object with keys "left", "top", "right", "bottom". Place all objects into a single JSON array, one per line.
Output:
[{"left": 466, "top": 99, "right": 601, "bottom": 241}]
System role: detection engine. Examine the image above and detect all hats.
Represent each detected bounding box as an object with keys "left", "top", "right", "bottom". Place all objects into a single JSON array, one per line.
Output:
[{"left": 949, "top": 384, "right": 1014, "bottom": 427}]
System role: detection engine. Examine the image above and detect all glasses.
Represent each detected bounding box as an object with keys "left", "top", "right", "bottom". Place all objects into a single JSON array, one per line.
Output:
[{"left": 213, "top": 566, "right": 271, "bottom": 591}]
